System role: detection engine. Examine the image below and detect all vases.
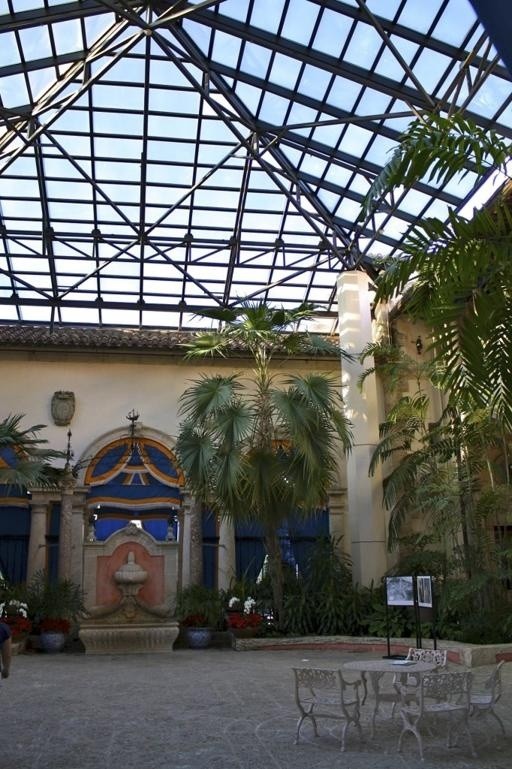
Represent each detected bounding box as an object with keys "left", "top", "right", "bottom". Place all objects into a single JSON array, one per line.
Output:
[
  {"left": 184, "top": 624, "right": 210, "bottom": 649},
  {"left": 231, "top": 626, "right": 257, "bottom": 638},
  {"left": 41, "top": 631, "right": 65, "bottom": 653}
]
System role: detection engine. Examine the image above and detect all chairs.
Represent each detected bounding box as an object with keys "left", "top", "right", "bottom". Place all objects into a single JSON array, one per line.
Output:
[{"left": 291, "top": 646, "right": 510, "bottom": 764}]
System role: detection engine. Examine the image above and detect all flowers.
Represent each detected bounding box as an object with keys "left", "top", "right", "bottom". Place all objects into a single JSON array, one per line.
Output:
[
  {"left": 227, "top": 611, "right": 261, "bottom": 629},
  {"left": 0, "top": 611, "right": 70, "bottom": 642},
  {"left": 183, "top": 614, "right": 209, "bottom": 627}
]
